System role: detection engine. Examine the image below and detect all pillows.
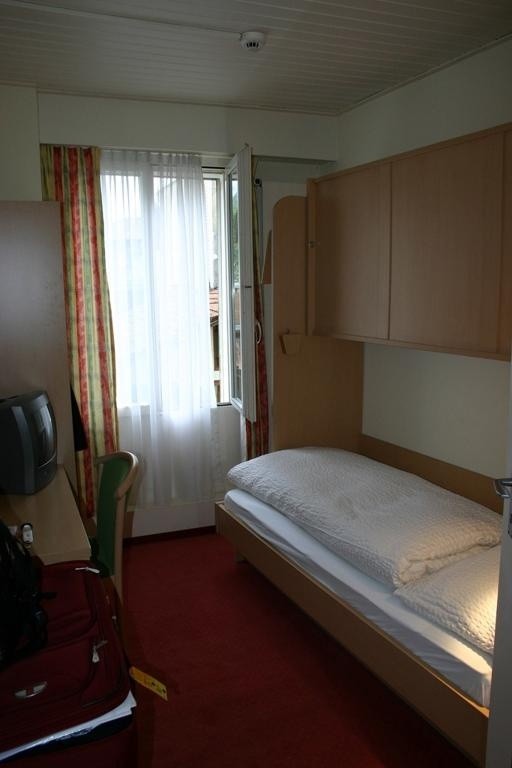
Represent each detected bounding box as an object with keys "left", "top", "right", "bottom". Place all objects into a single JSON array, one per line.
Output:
[{"left": 394, "top": 544, "right": 504, "bottom": 653}]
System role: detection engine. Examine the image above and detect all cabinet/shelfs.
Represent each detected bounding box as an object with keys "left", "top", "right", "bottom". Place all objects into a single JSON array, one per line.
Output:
[{"left": 305, "top": 126, "right": 509, "bottom": 363}]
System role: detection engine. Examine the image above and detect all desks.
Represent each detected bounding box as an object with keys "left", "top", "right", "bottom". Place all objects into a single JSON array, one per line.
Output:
[{"left": 2, "top": 465, "right": 92, "bottom": 568}]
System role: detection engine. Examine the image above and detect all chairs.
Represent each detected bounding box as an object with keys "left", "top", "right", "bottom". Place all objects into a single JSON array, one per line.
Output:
[{"left": 82, "top": 450, "right": 143, "bottom": 605}]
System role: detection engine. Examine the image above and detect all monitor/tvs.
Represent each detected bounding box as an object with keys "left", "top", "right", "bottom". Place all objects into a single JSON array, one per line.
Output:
[{"left": 0, "top": 389, "right": 58, "bottom": 494}]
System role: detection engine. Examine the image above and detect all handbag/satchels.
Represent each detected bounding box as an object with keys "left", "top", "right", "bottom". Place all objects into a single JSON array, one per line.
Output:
[{"left": 2, "top": 553, "right": 47, "bottom": 667}]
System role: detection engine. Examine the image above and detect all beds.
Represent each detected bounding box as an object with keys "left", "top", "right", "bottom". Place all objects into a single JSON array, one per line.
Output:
[{"left": 210, "top": 445, "right": 510, "bottom": 768}]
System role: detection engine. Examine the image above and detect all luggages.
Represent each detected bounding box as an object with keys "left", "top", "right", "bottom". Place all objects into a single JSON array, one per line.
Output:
[{"left": 1, "top": 559, "right": 138, "bottom": 767}]
